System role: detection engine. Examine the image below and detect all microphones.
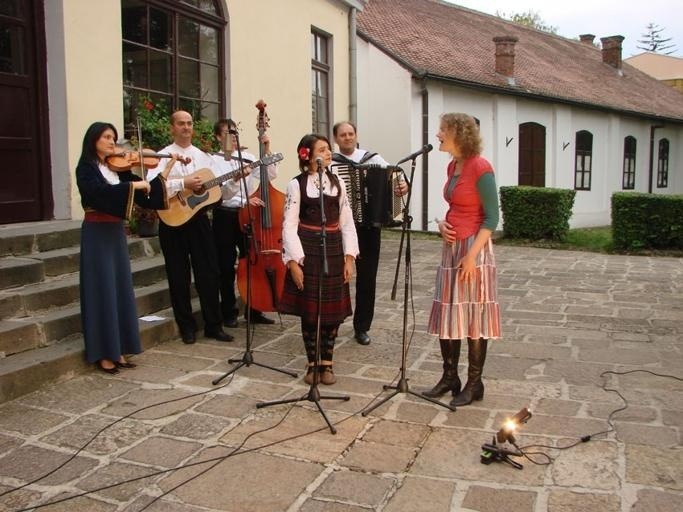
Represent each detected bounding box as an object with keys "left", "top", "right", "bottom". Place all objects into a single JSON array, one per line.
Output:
[
  {"left": 398, "top": 144, "right": 433, "bottom": 163},
  {"left": 228, "top": 129, "right": 238, "bottom": 134},
  {"left": 315, "top": 157, "right": 322, "bottom": 171}
]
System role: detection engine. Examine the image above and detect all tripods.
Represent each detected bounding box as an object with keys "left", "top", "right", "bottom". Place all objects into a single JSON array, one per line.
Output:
[
  {"left": 360, "top": 164, "right": 458, "bottom": 417},
  {"left": 210, "top": 135, "right": 299, "bottom": 385},
  {"left": 256, "top": 172, "right": 350, "bottom": 436}
]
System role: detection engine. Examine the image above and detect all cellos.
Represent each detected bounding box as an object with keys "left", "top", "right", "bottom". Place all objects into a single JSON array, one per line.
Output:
[{"left": 238, "top": 99, "right": 287, "bottom": 312}]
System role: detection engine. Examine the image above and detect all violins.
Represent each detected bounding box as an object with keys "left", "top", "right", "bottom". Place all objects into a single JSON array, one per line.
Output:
[{"left": 107, "top": 148, "right": 190, "bottom": 171}]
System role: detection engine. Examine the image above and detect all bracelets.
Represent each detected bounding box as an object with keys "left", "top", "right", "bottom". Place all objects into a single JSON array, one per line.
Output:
[{"left": 158, "top": 174, "right": 167, "bottom": 182}]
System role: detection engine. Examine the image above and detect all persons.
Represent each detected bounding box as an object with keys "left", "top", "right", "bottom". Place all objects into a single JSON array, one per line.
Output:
[
  {"left": 421, "top": 113, "right": 502, "bottom": 406},
  {"left": 327, "top": 122, "right": 410, "bottom": 344},
  {"left": 146, "top": 112, "right": 251, "bottom": 345},
  {"left": 278, "top": 134, "right": 361, "bottom": 385},
  {"left": 210, "top": 118, "right": 278, "bottom": 327},
  {"left": 76, "top": 122, "right": 177, "bottom": 376}
]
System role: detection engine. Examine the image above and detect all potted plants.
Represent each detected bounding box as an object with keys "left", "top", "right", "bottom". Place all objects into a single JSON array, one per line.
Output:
[{"left": 133, "top": 204, "right": 159, "bottom": 237}]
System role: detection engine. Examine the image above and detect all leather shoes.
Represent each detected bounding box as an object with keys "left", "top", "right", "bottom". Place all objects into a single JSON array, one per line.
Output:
[
  {"left": 244, "top": 309, "right": 275, "bottom": 325},
  {"left": 353, "top": 325, "right": 372, "bottom": 346},
  {"left": 112, "top": 355, "right": 137, "bottom": 370},
  {"left": 320, "top": 363, "right": 335, "bottom": 385},
  {"left": 204, "top": 326, "right": 235, "bottom": 342},
  {"left": 224, "top": 315, "right": 239, "bottom": 328},
  {"left": 179, "top": 327, "right": 195, "bottom": 344},
  {"left": 96, "top": 355, "right": 120, "bottom": 375},
  {"left": 305, "top": 360, "right": 320, "bottom": 384}
]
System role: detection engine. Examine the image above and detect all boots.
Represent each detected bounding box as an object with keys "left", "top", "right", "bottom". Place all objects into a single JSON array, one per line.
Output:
[
  {"left": 420, "top": 330, "right": 461, "bottom": 398},
  {"left": 450, "top": 335, "right": 488, "bottom": 407}
]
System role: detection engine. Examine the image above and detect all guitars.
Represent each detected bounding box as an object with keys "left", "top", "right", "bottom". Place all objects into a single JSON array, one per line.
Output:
[{"left": 157, "top": 155, "right": 283, "bottom": 228}]
